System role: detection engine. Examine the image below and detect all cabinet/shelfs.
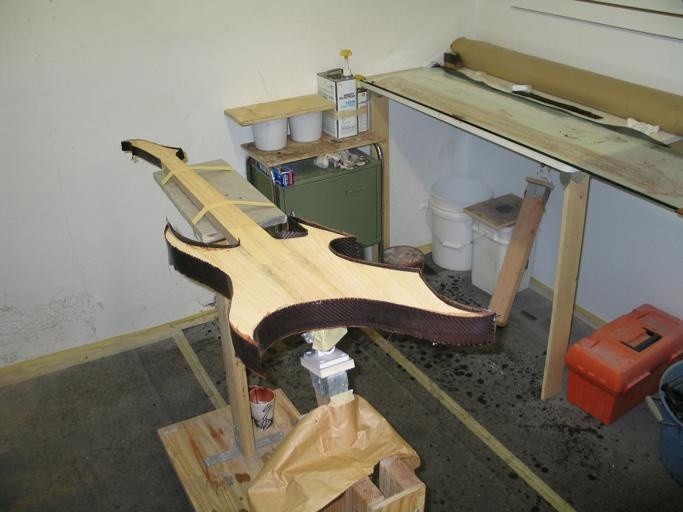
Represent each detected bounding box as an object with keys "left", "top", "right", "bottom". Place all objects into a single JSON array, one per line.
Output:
[{"left": 246, "top": 148, "right": 382, "bottom": 249}]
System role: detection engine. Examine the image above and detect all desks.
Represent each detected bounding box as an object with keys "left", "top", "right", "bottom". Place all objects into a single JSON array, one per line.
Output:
[{"left": 356, "top": 62, "right": 683, "bottom": 403}]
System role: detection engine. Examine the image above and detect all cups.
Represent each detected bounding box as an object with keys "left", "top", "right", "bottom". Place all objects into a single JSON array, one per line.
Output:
[{"left": 248, "top": 387, "right": 275, "bottom": 433}]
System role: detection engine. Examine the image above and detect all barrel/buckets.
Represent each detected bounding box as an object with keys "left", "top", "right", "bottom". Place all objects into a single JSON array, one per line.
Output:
[
  {"left": 644, "top": 363, "right": 681, "bottom": 481},
  {"left": 427, "top": 179, "right": 493, "bottom": 272}
]
